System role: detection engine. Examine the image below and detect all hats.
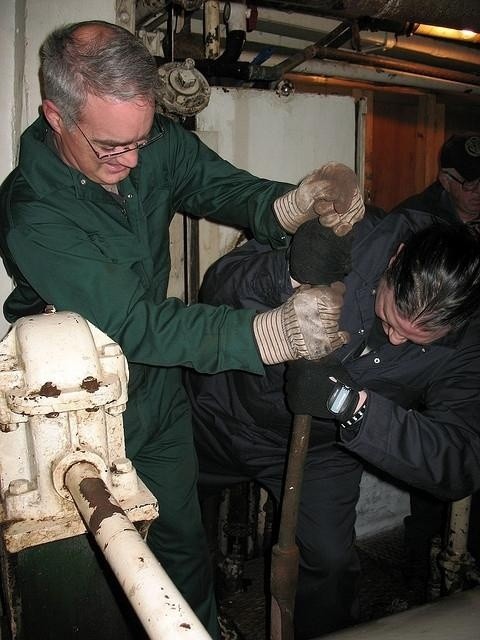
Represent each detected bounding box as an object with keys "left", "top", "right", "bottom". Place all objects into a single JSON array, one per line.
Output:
[{"left": 438, "top": 129, "right": 480, "bottom": 181}]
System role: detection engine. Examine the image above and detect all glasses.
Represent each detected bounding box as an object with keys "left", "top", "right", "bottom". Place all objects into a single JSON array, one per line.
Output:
[
  {"left": 60, "top": 94, "right": 168, "bottom": 165},
  {"left": 440, "top": 169, "right": 480, "bottom": 192}
]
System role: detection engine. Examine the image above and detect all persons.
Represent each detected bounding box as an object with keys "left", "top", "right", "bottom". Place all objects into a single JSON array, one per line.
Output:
[
  {"left": 189, "top": 134, "right": 479, "bottom": 640},
  {"left": 0, "top": 19, "right": 365, "bottom": 640}
]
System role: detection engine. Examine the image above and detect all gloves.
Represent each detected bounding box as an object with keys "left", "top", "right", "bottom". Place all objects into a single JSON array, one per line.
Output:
[
  {"left": 284, "top": 217, "right": 363, "bottom": 283},
  {"left": 273, "top": 160, "right": 368, "bottom": 239},
  {"left": 252, "top": 280, "right": 353, "bottom": 366},
  {"left": 281, "top": 352, "right": 361, "bottom": 425}
]
